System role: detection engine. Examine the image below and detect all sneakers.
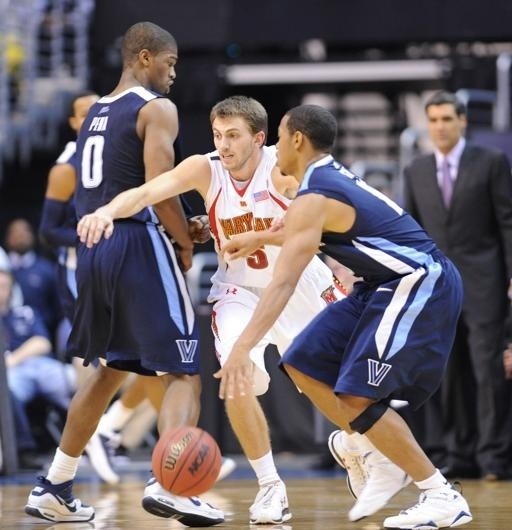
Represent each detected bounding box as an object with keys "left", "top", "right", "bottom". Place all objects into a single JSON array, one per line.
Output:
[
  {"left": 86, "top": 416, "right": 122, "bottom": 484},
  {"left": 251, "top": 483, "right": 291, "bottom": 526},
  {"left": 328, "top": 428, "right": 367, "bottom": 497},
  {"left": 143, "top": 475, "right": 224, "bottom": 527},
  {"left": 24, "top": 476, "right": 94, "bottom": 521},
  {"left": 385, "top": 481, "right": 472, "bottom": 530},
  {"left": 346, "top": 458, "right": 412, "bottom": 522}
]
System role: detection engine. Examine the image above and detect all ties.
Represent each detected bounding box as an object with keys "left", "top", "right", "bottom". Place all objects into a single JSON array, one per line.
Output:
[{"left": 441, "top": 160, "right": 453, "bottom": 208}]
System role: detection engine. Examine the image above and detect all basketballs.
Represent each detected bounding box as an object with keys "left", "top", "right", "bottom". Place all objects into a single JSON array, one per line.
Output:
[{"left": 151, "top": 426, "right": 221, "bottom": 495}]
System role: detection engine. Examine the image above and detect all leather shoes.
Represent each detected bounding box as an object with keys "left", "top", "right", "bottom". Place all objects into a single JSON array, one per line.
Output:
[{"left": 442, "top": 463, "right": 477, "bottom": 479}]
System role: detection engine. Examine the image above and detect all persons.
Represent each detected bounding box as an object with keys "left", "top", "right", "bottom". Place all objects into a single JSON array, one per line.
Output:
[
  {"left": 1, "top": 16, "right": 511, "bottom": 483},
  {"left": 26, "top": 21, "right": 475, "bottom": 530}
]
